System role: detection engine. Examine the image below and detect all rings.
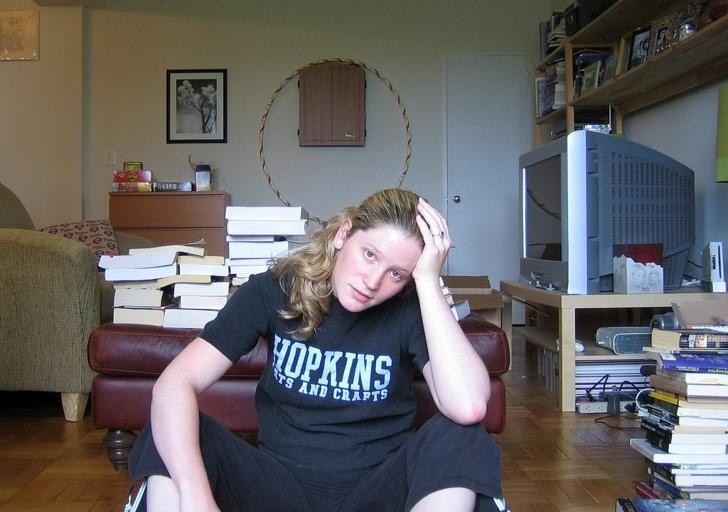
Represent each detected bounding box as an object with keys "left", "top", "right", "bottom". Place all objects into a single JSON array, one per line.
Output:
[{"left": 432, "top": 231, "right": 444, "bottom": 238}]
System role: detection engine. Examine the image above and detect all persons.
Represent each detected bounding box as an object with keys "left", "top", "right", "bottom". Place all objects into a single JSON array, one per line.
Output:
[{"left": 124, "top": 190, "right": 503, "bottom": 512}]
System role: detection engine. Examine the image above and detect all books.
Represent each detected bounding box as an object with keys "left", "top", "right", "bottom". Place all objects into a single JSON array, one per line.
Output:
[
  {"left": 531, "top": 59, "right": 566, "bottom": 118},
  {"left": 96, "top": 204, "right": 310, "bottom": 329},
  {"left": 616, "top": 326, "right": 728, "bottom": 512},
  {"left": 544, "top": 29, "right": 567, "bottom": 57},
  {"left": 437, "top": 276, "right": 471, "bottom": 322}
]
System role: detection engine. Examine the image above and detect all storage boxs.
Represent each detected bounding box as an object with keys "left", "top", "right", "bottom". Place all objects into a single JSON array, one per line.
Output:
[{"left": 441, "top": 274, "right": 502, "bottom": 330}]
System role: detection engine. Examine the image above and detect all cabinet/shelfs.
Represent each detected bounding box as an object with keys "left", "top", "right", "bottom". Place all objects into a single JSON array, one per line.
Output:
[
  {"left": 109, "top": 191, "right": 231, "bottom": 258},
  {"left": 532, "top": 0, "right": 728, "bottom": 150},
  {"left": 297, "top": 64, "right": 368, "bottom": 147},
  {"left": 499, "top": 280, "right": 728, "bottom": 413}
]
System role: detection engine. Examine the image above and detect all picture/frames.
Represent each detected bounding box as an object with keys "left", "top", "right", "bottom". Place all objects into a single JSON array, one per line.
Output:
[
  {"left": 163, "top": 68, "right": 228, "bottom": 144},
  {"left": 0, "top": 7, "right": 40, "bottom": 62}
]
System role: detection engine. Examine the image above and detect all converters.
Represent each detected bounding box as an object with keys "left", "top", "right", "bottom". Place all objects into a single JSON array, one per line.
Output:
[{"left": 607, "top": 398, "right": 620, "bottom": 417}]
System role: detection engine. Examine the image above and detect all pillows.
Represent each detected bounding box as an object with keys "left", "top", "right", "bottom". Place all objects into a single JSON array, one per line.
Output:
[{"left": 37, "top": 219, "right": 121, "bottom": 270}]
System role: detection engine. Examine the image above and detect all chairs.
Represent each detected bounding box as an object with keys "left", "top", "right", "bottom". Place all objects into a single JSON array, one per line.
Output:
[{"left": 1, "top": 184, "right": 163, "bottom": 421}]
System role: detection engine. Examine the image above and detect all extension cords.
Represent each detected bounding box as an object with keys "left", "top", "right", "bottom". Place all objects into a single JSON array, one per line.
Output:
[{"left": 578, "top": 397, "right": 641, "bottom": 414}]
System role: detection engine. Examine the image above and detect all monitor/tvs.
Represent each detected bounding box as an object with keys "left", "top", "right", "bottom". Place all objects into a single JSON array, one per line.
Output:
[{"left": 519, "top": 129, "right": 695, "bottom": 295}]
